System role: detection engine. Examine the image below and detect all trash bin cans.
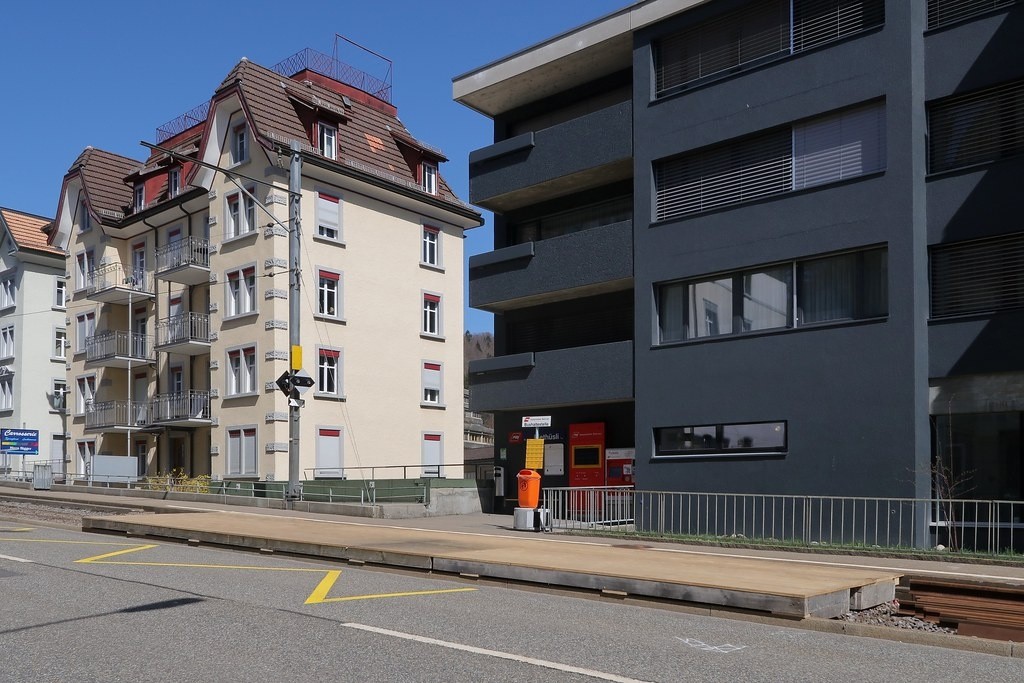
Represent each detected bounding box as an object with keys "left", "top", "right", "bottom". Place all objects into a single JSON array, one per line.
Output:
[
  {"left": 493, "top": 465, "right": 504, "bottom": 497},
  {"left": 516, "top": 469, "right": 541, "bottom": 508}
]
